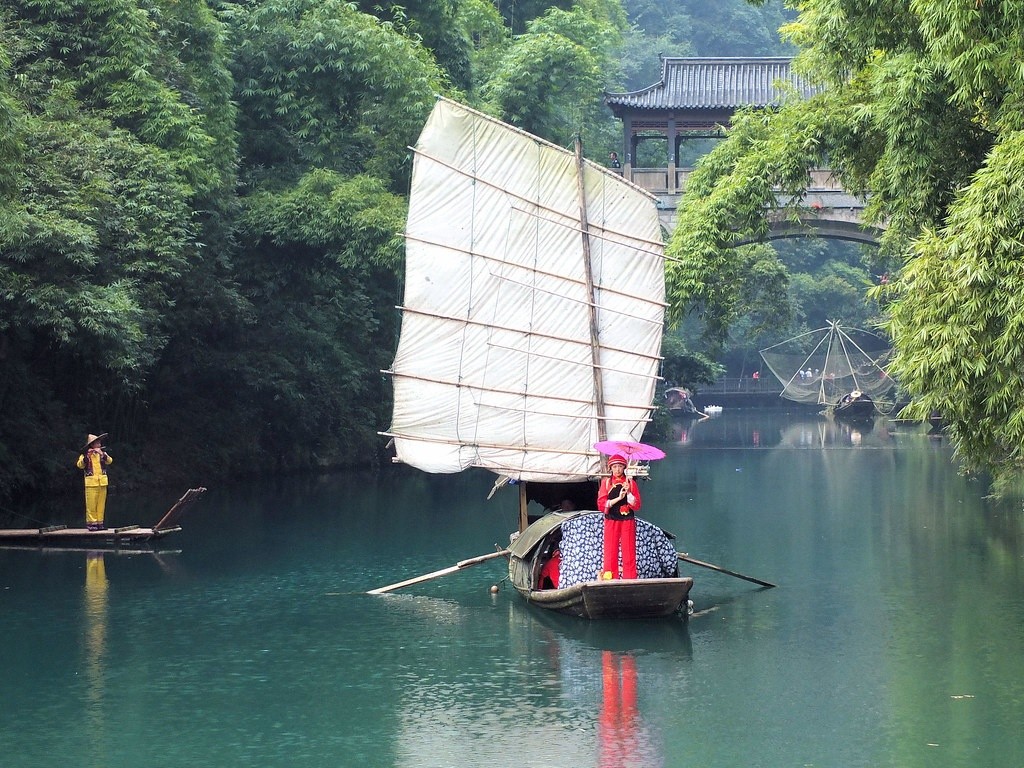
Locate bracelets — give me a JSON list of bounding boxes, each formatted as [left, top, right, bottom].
[[610, 500, 613, 507]]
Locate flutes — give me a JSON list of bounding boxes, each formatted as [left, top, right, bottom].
[[88, 446, 106, 452]]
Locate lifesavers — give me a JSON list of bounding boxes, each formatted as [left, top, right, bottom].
[[851, 391, 861, 398]]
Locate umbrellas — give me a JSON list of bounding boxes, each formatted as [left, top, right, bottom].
[[593, 440, 667, 492]]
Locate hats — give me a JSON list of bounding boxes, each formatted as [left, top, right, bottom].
[[608, 454, 627, 469], [81, 433, 108, 449]]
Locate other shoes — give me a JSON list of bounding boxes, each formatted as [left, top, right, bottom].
[[88, 525, 98, 531], [98, 524, 108, 530]]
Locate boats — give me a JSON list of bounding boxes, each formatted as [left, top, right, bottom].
[[664, 386, 694, 417], [832, 392, 876, 420], [0, 486, 207, 554]]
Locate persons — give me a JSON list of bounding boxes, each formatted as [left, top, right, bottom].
[[77, 433, 113, 532], [845, 391, 864, 402], [595, 648, 640, 768], [752, 371, 759, 392], [596, 454, 641, 579], [538, 534, 562, 589], [792, 368, 836, 391], [667, 388, 688, 409]]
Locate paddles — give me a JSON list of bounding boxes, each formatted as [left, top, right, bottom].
[[367, 549, 511, 594], [695, 410, 709, 416], [678, 555, 776, 587], [697, 416, 710, 423]]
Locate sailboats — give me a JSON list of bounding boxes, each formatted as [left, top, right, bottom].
[[377, 92, 693, 621]]
